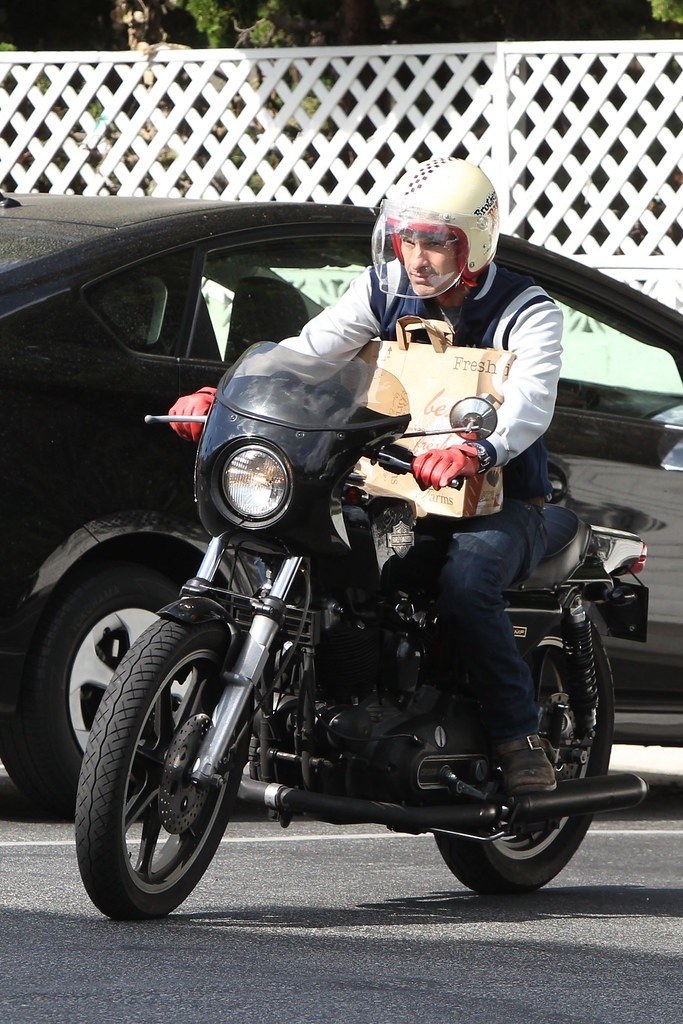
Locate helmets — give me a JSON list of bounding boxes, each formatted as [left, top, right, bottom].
[[382, 157, 499, 281]]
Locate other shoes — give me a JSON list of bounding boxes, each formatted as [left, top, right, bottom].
[[497, 734, 557, 795]]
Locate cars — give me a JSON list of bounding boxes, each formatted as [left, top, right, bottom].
[[0, 169, 683, 816]]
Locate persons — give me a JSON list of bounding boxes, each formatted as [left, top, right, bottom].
[[164, 160, 564, 793]]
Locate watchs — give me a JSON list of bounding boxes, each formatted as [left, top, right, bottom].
[[467, 442, 490, 475]]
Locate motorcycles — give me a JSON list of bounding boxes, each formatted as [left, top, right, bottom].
[[75, 341, 654, 929]]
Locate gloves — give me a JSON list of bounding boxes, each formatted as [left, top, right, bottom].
[[168, 386, 217, 442], [412, 438, 497, 491]]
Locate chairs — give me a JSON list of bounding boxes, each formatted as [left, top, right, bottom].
[[105, 276, 168, 347], [223, 278, 310, 363]]
